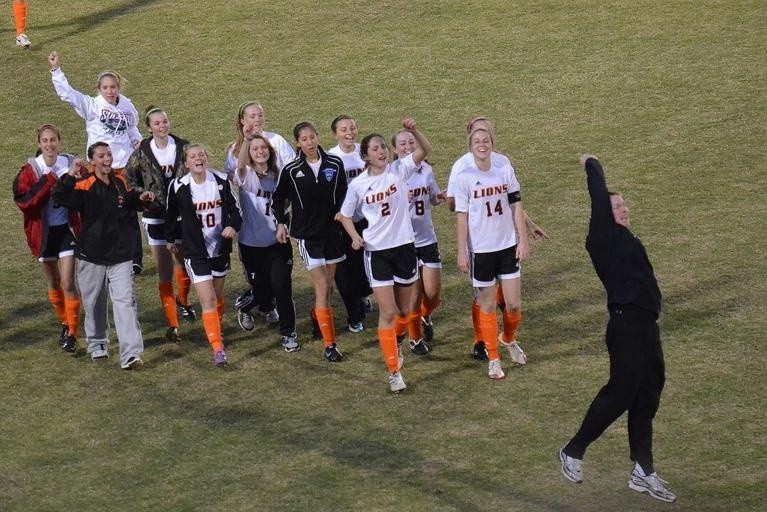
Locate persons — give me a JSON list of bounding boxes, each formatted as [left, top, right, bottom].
[[272, 122, 349, 362], [163, 144, 243, 367], [325, 114, 374, 333], [224, 100, 297, 326], [390, 130, 446, 369], [124, 105, 197, 343], [48, 50, 143, 275], [339, 111, 433, 392], [454, 129, 529, 380], [446, 116, 550, 360], [232, 114, 301, 353], [12, 124, 81, 352], [559, 152, 678, 504], [52, 142, 156, 370], [12, 0, 32, 47]]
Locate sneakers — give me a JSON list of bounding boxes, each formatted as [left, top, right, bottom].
[[348, 321, 364, 333], [167, 327, 178, 340], [260, 308, 279, 326], [628, 469, 677, 503], [14, 35, 31, 47], [388, 372, 407, 392], [236, 296, 255, 331], [311, 310, 323, 341], [62, 336, 79, 353], [133, 269, 142, 274], [213, 349, 227, 367], [488, 359, 504, 379], [558, 442, 584, 483], [474, 341, 488, 361], [421, 315, 434, 342], [91, 345, 109, 361], [409, 338, 428, 355], [362, 297, 372, 309], [324, 343, 345, 363], [59, 326, 68, 345], [280, 332, 300, 352], [176, 296, 198, 324], [120, 358, 143, 369], [498, 332, 528, 364], [398, 352, 403, 370]]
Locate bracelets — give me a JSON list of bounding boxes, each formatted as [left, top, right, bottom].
[[243, 137, 253, 143]]
